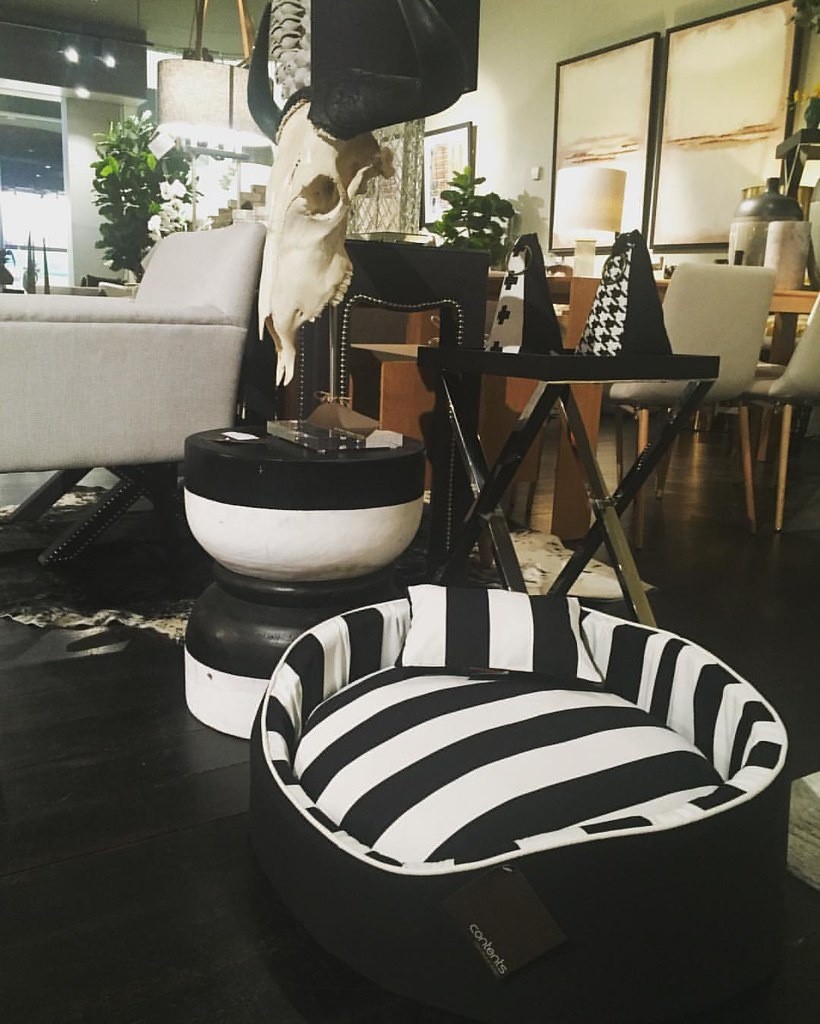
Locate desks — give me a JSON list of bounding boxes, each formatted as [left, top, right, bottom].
[[177, 422, 431, 740], [416, 347, 722, 631]]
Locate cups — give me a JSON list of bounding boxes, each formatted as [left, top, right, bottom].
[[763, 221, 813, 289]]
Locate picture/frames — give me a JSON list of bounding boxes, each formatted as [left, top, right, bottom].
[[649, 1, 802, 251], [420, 121, 474, 230], [547, 31, 659, 258]]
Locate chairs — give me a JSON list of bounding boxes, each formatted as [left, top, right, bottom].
[[731, 288, 820, 531], [3, 215, 262, 565], [608, 259, 778, 553]]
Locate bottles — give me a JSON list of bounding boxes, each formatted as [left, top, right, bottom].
[[728, 177, 803, 268]]
